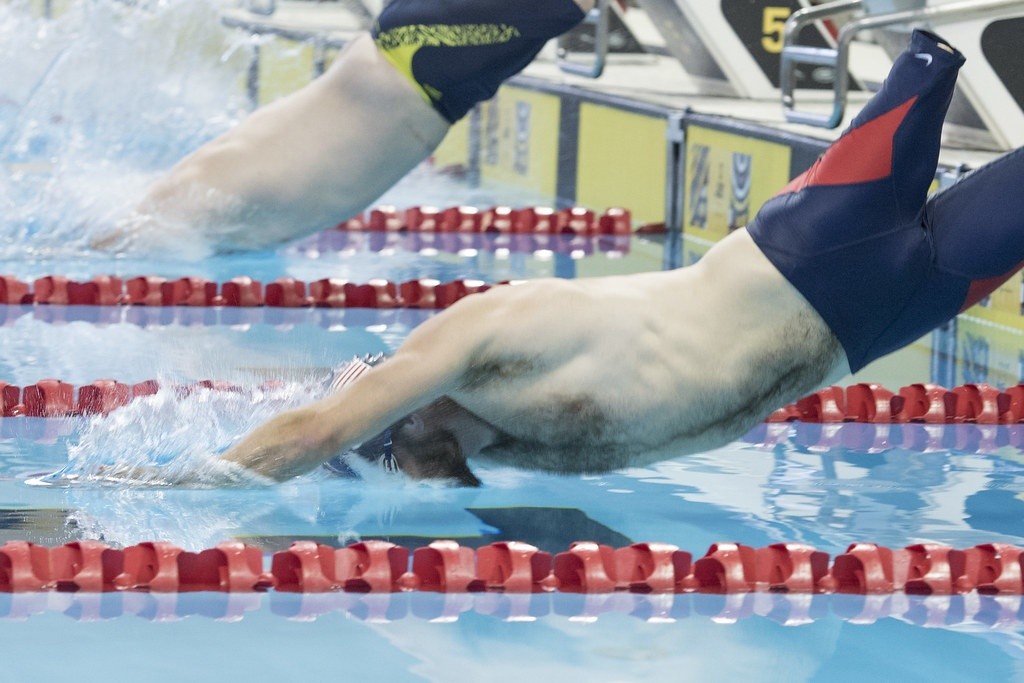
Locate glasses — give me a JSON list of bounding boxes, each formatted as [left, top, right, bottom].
[[377, 425, 401, 481]]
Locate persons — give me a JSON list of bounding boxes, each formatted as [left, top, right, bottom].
[[82, 0, 598, 262], [202, 28, 1023, 481]]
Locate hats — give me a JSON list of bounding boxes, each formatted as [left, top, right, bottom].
[[317, 355, 383, 479]]
